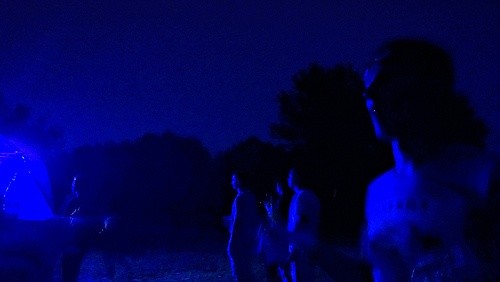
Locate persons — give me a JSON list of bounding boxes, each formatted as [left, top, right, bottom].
[[223, 165, 264, 282], [358, 36, 499, 282], [275, 178, 292, 221], [278, 163, 321, 282]]
